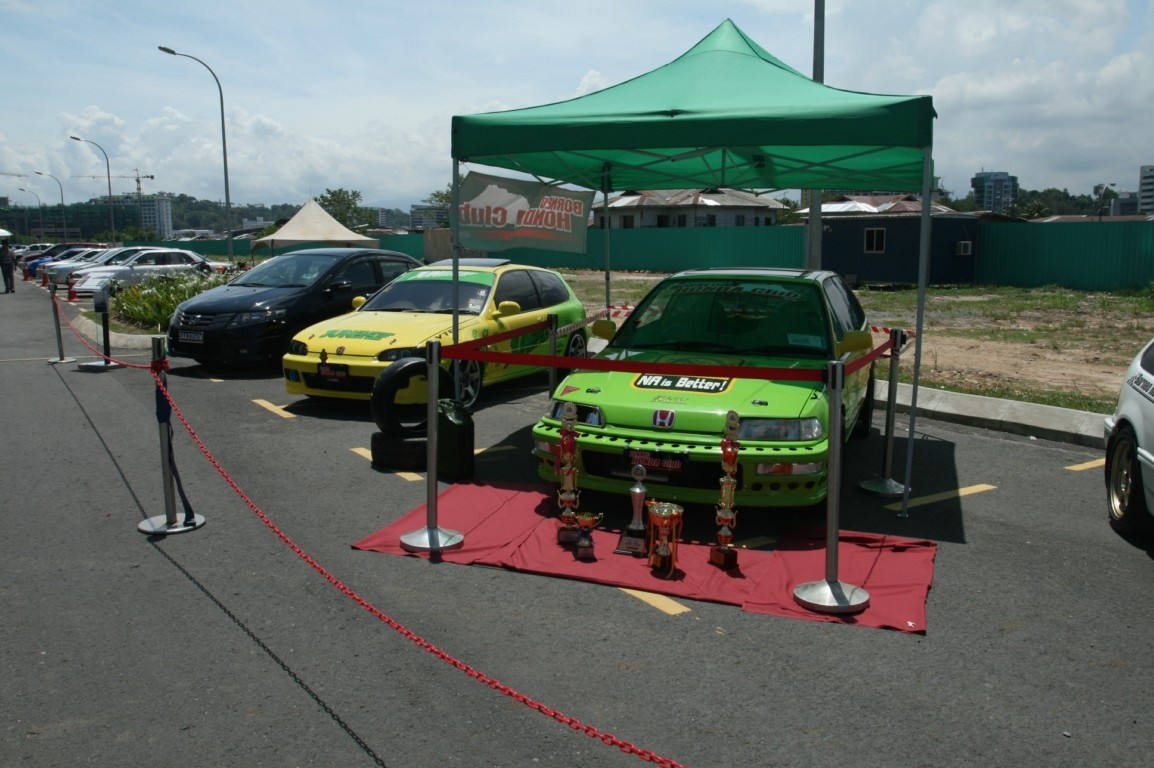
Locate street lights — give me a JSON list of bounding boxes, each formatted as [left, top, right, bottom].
[[156, 44, 238, 268], [70, 135, 117, 249], [34, 171, 69, 244], [17, 186, 44, 247]]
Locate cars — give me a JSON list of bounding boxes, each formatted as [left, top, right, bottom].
[[1099, 335, 1153, 537], [5, 239, 163, 283], [278, 255, 591, 419], [166, 247, 435, 377], [532, 264, 877, 521], [67, 249, 238, 298]]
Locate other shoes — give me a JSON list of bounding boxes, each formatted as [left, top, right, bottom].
[[11, 289, 15, 293], [5, 289, 10, 293]]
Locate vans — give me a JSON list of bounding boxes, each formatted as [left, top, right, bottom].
[[172, 229, 214, 242]]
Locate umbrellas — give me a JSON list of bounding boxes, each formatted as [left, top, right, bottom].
[[0, 229, 13, 236]]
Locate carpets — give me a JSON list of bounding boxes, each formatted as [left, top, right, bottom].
[[351, 455, 938, 635]]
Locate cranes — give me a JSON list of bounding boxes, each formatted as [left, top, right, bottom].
[[72, 168, 154, 233]]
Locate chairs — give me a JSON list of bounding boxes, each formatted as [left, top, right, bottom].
[[665, 297, 724, 342], [762, 299, 826, 342]]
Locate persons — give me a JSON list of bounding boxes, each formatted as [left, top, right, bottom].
[[200, 262, 211, 275], [0, 240, 18, 294]]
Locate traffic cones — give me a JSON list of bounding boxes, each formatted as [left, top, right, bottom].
[[40, 265, 52, 286], [61, 270, 81, 302], [21, 262, 33, 280]]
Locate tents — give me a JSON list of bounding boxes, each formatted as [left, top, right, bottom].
[[451, 18, 939, 518], [251, 199, 380, 268]]
